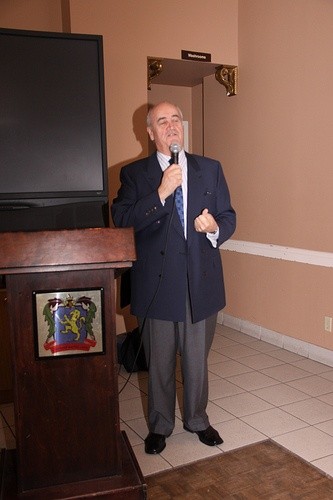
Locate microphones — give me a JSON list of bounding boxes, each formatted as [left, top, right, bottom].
[[169, 143, 180, 166]]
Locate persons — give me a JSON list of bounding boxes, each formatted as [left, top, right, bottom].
[[110, 101, 237, 454]]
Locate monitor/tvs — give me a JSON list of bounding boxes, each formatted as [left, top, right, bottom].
[[0, 28, 110, 211]]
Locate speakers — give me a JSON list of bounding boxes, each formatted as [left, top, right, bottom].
[[118, 327, 146, 372]]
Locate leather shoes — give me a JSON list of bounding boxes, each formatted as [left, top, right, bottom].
[[145, 431, 172, 454], [183, 424, 223, 446]]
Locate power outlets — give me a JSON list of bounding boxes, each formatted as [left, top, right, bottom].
[[324, 316, 332, 333]]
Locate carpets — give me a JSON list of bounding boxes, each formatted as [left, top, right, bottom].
[[144, 439, 333, 500]]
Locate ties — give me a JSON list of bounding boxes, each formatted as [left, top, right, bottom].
[[169, 159, 183, 230]]
